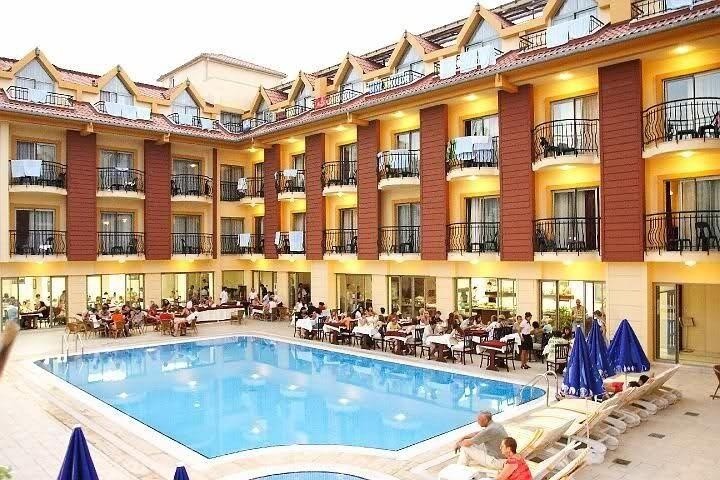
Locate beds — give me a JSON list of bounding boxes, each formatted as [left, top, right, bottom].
[[65, 308, 199, 338]]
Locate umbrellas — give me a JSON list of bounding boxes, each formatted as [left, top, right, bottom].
[[561, 319, 650, 446], [173, 467, 189, 480], [57, 427, 99, 480]]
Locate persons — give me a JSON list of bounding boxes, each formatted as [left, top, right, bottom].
[[387, 307, 552, 370], [460, 282, 497, 304], [455, 411, 534, 480], [342, 284, 387, 350], [188, 280, 215, 309], [571, 298, 605, 339], [220, 286, 234, 306], [147, 298, 195, 337], [543, 325, 572, 374], [290, 282, 330, 340], [241, 284, 283, 322], [86, 287, 144, 337], [2, 289, 66, 327]]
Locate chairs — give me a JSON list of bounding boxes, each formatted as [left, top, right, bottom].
[[479, 230, 499, 252], [37, 237, 54, 256], [400, 232, 414, 253], [125, 238, 137, 254], [181, 239, 193, 253], [437, 364, 682, 480], [536, 228, 558, 252], [345, 235, 357, 253], [667, 222, 720, 251], [37, 306, 50, 328], [230, 299, 291, 325], [293, 309, 571, 374]]
